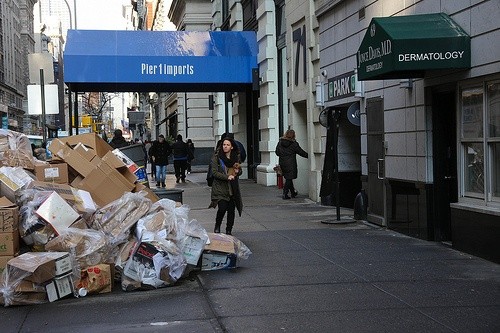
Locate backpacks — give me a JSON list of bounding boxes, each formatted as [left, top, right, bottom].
[[206, 159, 220, 186]]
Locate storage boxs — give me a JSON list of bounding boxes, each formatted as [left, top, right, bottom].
[[0, 129, 239, 307]]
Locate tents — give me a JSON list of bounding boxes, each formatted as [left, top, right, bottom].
[[63, 28, 259, 180]]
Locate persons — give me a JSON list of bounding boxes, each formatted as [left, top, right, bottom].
[[275, 130, 308, 200], [206, 132, 247, 236], [102, 129, 196, 188]]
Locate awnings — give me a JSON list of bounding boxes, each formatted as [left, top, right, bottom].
[[355, 11, 472, 82]]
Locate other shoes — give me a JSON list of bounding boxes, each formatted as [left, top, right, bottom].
[[226, 227, 231, 234], [214, 224, 220, 233], [282, 195, 290, 200], [162, 184, 166, 187], [156, 183, 160, 186], [292, 190, 297, 198], [176, 177, 180, 183], [182, 177, 185, 183]]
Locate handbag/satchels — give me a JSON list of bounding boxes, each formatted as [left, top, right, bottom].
[[273, 165, 283, 176]]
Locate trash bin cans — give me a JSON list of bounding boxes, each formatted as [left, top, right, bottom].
[[151, 188, 184, 206]]
